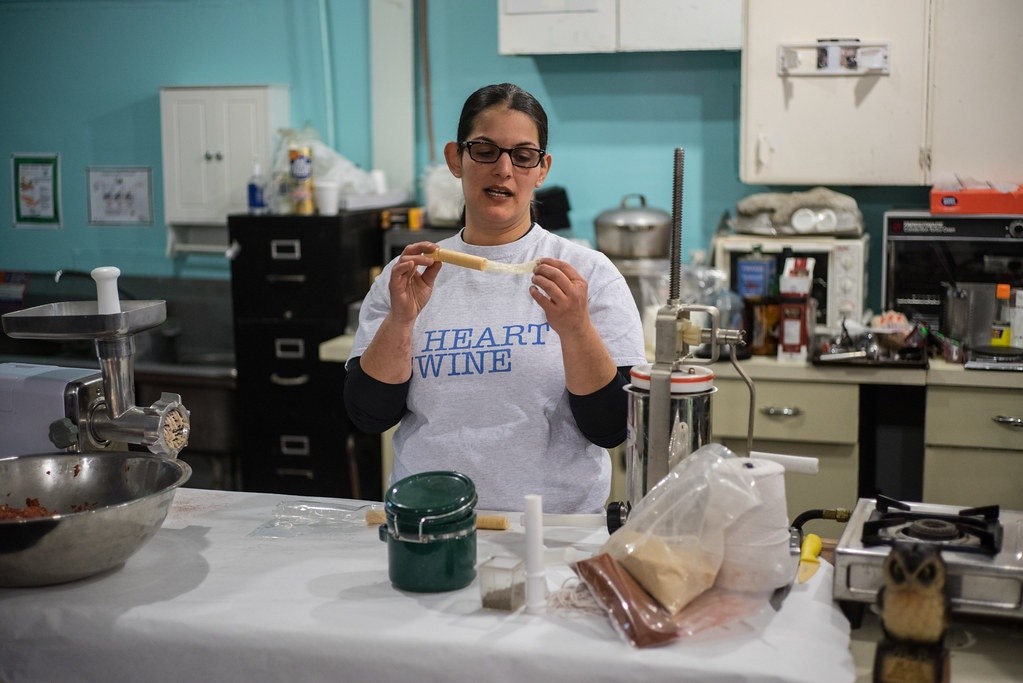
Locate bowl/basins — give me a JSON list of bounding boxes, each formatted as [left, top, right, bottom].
[[0, 452, 190, 587]]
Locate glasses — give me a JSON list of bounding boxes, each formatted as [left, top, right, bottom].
[[461, 140, 546, 168]]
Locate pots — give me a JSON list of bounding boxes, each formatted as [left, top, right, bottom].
[[593, 192, 671, 259], [939, 280, 1016, 356]]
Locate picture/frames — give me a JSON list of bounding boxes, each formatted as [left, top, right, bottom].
[[8, 151, 65, 232], [82, 164, 156, 229]]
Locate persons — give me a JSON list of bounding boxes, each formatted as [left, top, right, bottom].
[[343, 82, 647, 509]]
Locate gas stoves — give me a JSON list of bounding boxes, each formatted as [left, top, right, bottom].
[[833, 492, 1023, 630]]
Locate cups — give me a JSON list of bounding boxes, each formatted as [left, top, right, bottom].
[[311, 183, 339, 216]]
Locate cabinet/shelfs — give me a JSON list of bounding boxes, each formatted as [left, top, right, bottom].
[[159, 83, 290, 258], [494, 0, 1023, 196], [713, 375, 860, 543], [922, 385, 1023, 513], [228, 206, 411, 503]]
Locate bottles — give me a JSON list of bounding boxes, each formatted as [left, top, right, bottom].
[[990, 284, 1012, 347], [246, 164, 269, 216], [378, 470, 478, 594]]
